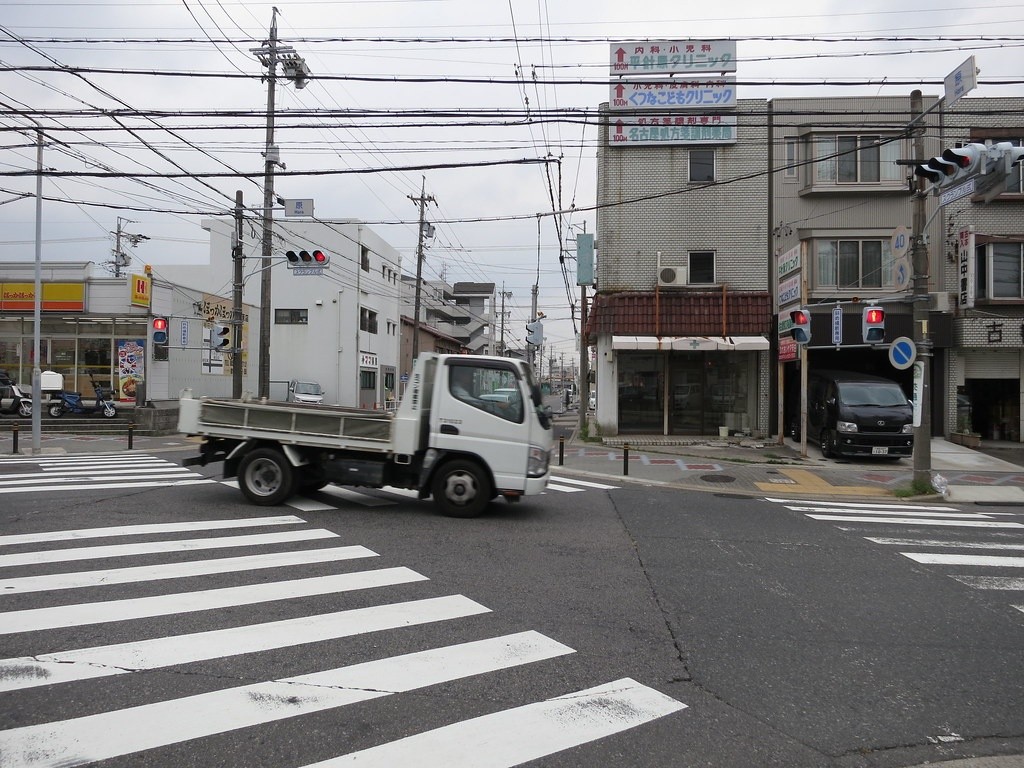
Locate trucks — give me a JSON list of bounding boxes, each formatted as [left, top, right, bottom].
[[182, 352, 570, 520]]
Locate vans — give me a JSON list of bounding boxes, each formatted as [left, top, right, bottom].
[[786, 368, 913, 460]]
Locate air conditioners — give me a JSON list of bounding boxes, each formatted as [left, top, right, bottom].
[[658, 265, 687, 286], [928, 292, 948, 311]]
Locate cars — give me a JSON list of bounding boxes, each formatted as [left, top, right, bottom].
[[477, 387, 518, 412]]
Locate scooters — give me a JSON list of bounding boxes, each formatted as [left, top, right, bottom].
[[43, 371, 117, 419], [0, 370, 32, 418]]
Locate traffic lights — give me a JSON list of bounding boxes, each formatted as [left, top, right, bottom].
[[1003, 141, 1024, 175], [862, 306, 886, 343], [152, 317, 168, 344], [285, 248, 329, 269], [213, 324, 229, 348], [790, 310, 811, 344], [914, 143, 989, 189]]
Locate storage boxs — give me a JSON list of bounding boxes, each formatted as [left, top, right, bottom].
[[41, 373, 64, 390]]
